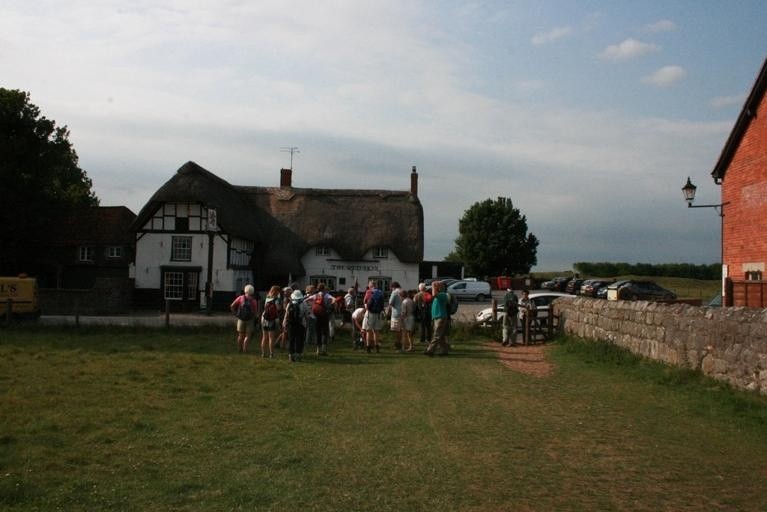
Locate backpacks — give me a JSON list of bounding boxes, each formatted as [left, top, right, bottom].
[[237, 295, 255, 321], [505, 294, 518, 317], [448, 293, 459, 315], [416, 293, 428, 312], [367, 290, 384, 314], [312, 293, 327, 317], [263, 297, 278, 321], [287, 301, 304, 327]]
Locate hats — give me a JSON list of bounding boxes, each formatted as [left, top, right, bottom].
[[336, 295, 344, 303], [290, 289, 304, 300]]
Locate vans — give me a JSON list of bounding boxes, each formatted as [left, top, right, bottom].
[[427, 278, 491, 303]]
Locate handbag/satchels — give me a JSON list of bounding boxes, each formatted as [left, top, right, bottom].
[[412, 299, 425, 322]]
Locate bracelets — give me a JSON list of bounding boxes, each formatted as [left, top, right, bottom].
[[231, 312, 236, 315]]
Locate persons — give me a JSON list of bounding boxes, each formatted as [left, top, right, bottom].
[[229, 283, 258, 355], [249, 285, 261, 341], [260, 277, 458, 364], [516, 289, 536, 345], [501, 287, 520, 347]]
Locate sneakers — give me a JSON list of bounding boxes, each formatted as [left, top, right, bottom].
[[261, 352, 274, 359], [287, 353, 304, 365], [420, 340, 449, 356], [502, 340, 515, 348], [316, 344, 329, 360], [393, 344, 414, 354]]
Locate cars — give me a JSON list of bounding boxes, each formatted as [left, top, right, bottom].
[[543, 275, 617, 298], [597, 279, 677, 303], [476, 292, 577, 334]]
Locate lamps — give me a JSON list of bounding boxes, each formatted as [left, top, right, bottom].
[[681, 176, 730, 217]]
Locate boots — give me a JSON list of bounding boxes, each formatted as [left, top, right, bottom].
[[352, 341, 380, 354]]
